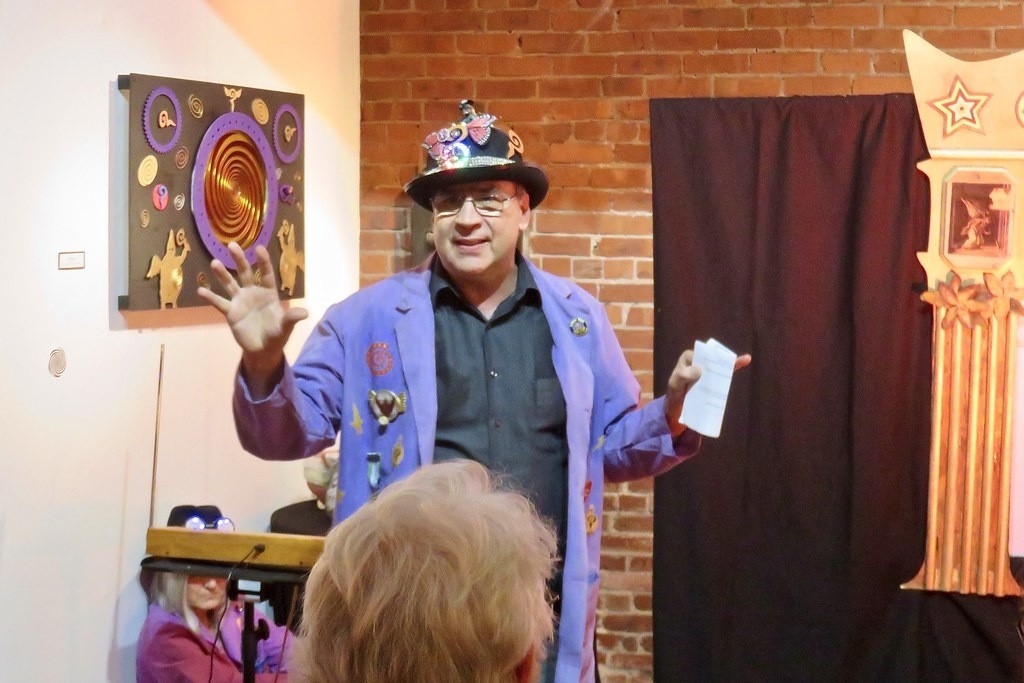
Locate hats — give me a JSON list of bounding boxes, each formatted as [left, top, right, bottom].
[[402, 100, 551, 212], [166, 505, 237, 531]]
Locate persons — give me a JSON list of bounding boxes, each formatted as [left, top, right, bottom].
[[196, 97, 764, 683], [295, 459, 558, 683], [134, 504, 294, 683]]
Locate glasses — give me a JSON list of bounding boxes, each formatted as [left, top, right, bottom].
[[427, 186, 518, 216], [183, 517, 239, 535]]
